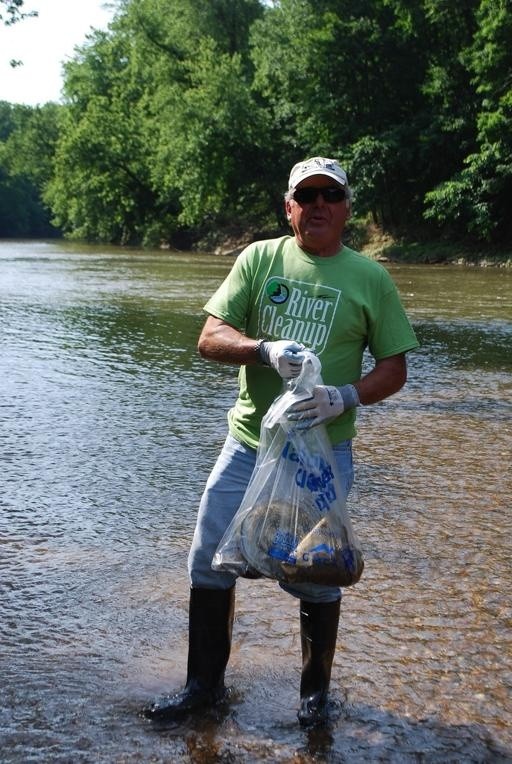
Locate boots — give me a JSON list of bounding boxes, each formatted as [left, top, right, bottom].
[[298, 600, 340, 724], [140, 585, 237, 722]]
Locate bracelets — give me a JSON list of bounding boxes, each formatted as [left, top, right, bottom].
[[254, 337, 270, 368]]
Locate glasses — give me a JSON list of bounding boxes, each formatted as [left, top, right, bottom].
[[289, 184, 351, 205]]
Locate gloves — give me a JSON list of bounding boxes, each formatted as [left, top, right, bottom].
[[288, 382, 360, 430], [260, 340, 305, 379]]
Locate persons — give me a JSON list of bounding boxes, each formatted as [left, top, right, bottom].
[[139, 156, 422, 730]]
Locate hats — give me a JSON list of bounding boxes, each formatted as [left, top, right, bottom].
[[288, 156, 351, 191]]
